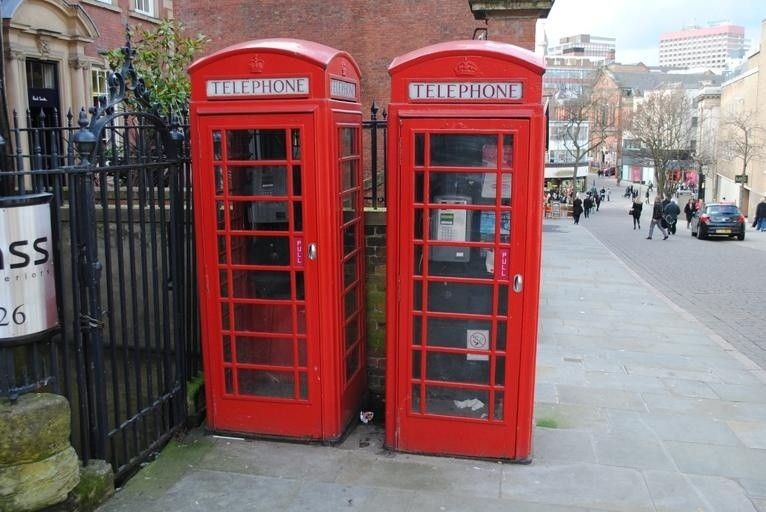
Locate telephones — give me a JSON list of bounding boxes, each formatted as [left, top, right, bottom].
[[239, 166, 297, 224], [417, 195, 473, 263]]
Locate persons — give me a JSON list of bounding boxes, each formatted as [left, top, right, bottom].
[[543, 165, 766, 232], [646, 196, 669, 240]]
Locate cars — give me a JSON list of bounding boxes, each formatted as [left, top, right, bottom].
[[691, 204, 746, 241], [603, 166, 614, 177]]
